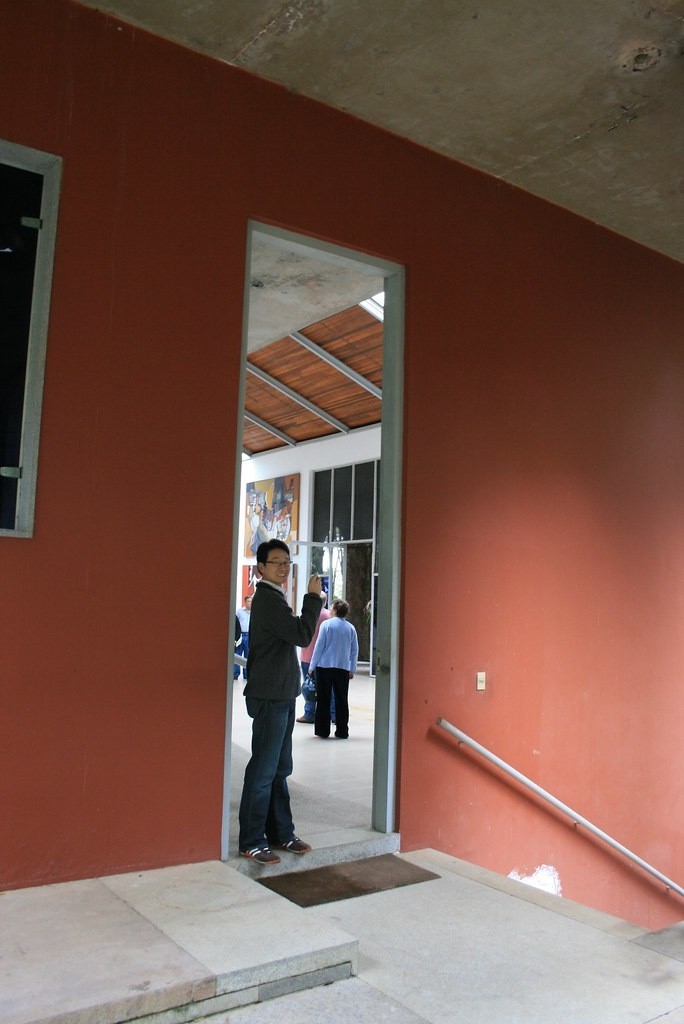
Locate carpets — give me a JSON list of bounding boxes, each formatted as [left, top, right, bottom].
[[256, 854, 442, 908]]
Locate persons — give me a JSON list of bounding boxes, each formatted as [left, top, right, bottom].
[[363, 601, 371, 624], [239, 539, 324, 864], [296, 590, 335, 723], [233, 595, 251, 680], [309, 600, 358, 739]]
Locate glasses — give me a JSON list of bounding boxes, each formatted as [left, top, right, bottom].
[[261, 559, 294, 568]]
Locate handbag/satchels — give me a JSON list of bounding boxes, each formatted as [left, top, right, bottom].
[[302, 673, 318, 702]]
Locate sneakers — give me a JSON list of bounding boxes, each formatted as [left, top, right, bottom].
[[239, 844, 280, 864], [268, 835, 311, 853]]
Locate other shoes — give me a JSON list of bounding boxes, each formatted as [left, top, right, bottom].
[[234, 675, 238, 679], [296, 715, 314, 724]]
